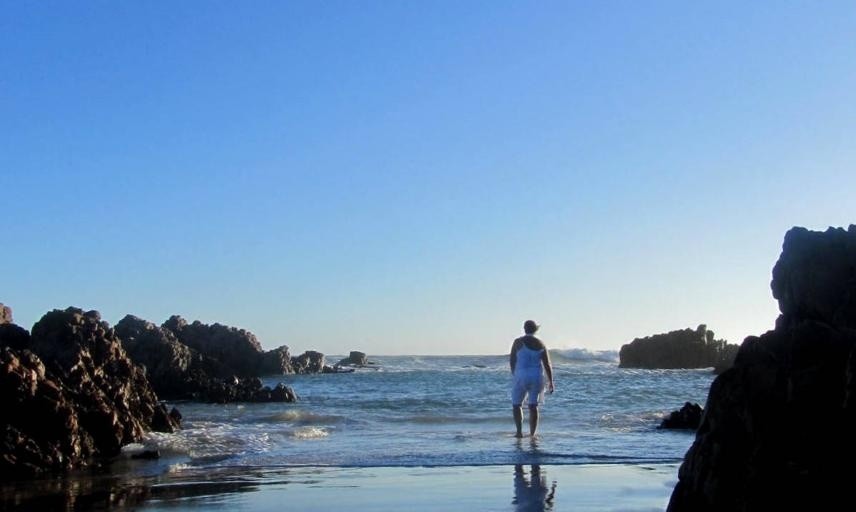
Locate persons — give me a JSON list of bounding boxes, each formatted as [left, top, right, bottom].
[[509, 319, 554, 438], [512, 437, 558, 512]]
